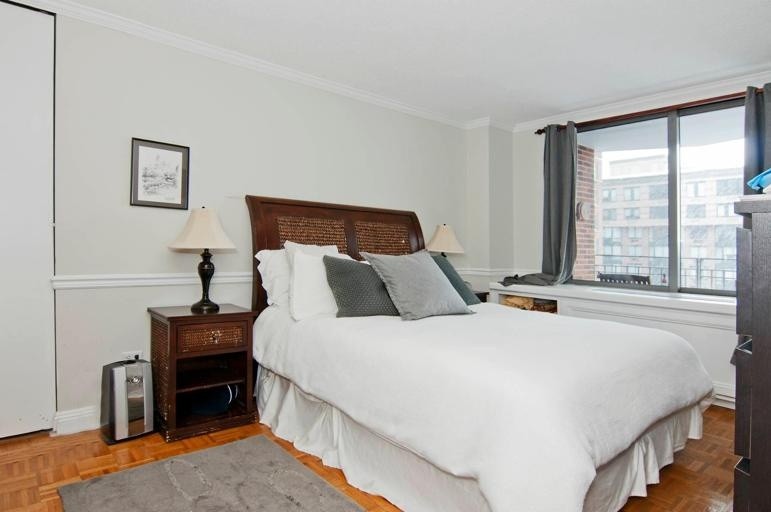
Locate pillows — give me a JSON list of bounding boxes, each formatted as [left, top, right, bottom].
[[254, 245, 340, 308], [322, 255, 401, 319], [429, 254, 484, 306], [284, 239, 372, 322], [358, 247, 478, 322]]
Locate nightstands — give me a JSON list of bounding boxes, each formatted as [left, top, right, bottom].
[[143, 301, 269, 444]]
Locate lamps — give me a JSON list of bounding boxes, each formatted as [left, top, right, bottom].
[[164, 205, 239, 314], [424, 223, 467, 261]]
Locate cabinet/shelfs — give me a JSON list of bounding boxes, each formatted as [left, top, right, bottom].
[[727, 196, 770, 512]]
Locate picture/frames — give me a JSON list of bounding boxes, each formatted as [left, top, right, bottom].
[[129, 136, 191, 209]]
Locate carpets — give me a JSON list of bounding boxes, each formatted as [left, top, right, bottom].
[[54, 433, 367, 512]]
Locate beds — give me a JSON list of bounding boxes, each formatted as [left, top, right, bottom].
[[245, 193, 716, 512]]
